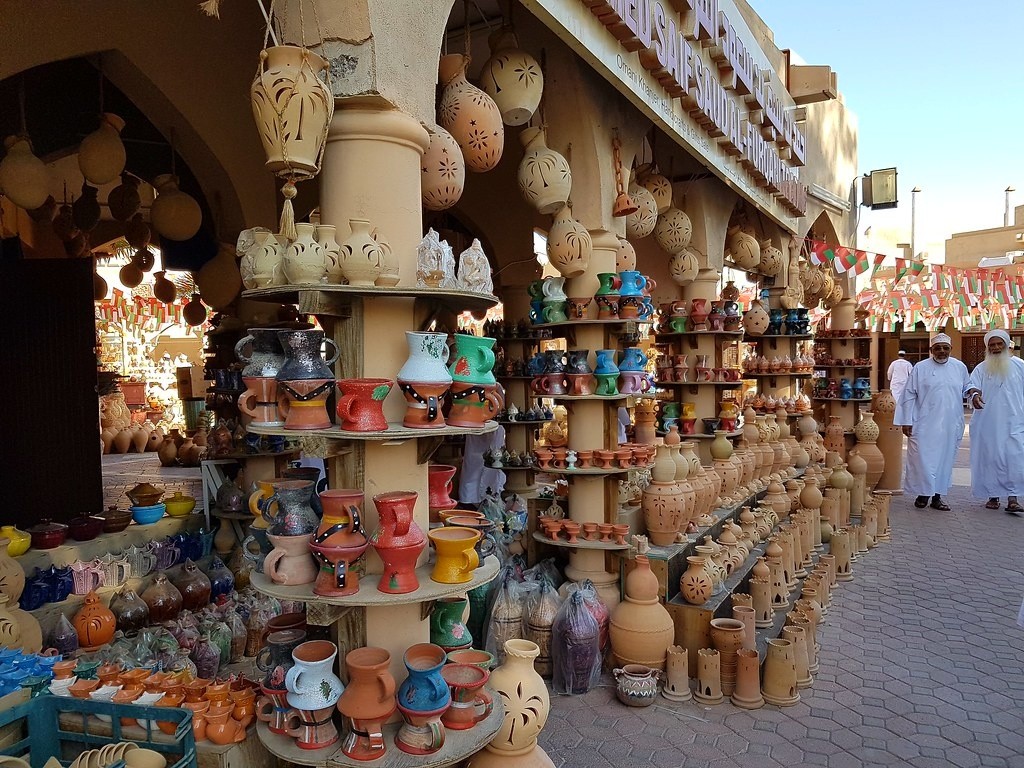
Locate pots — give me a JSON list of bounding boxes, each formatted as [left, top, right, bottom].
[[0, 482, 194, 554]]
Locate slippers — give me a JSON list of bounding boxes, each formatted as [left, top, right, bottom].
[[986, 500, 1000, 509], [930, 496, 950, 511], [914, 496, 930, 508], [1005, 500, 1024, 512]]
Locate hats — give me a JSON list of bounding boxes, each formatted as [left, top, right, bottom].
[[1009, 340, 1015, 348], [931, 333, 951, 347], [984, 330, 1010, 346], [899, 351, 905, 354]]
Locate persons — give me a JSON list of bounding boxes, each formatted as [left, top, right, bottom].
[[891, 333, 983, 511], [967, 328, 1024, 513], [886, 350, 914, 405]]
